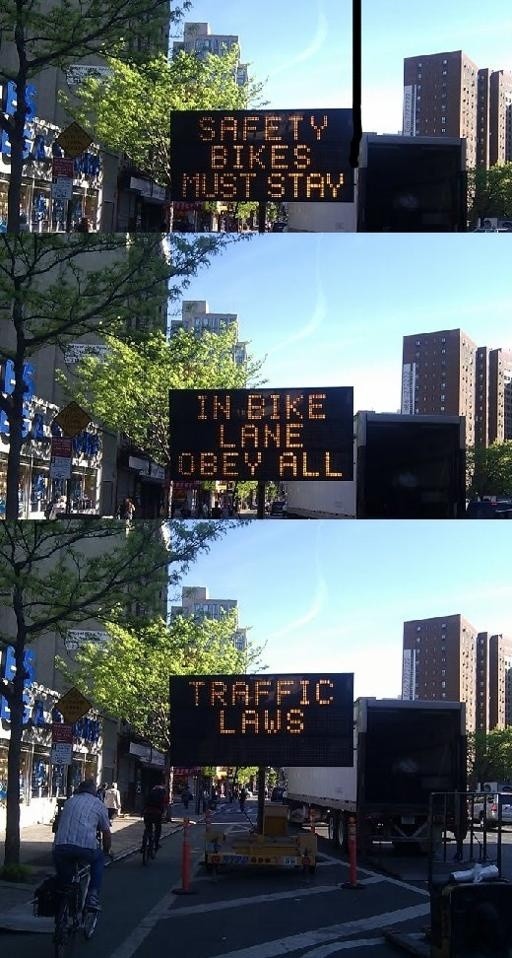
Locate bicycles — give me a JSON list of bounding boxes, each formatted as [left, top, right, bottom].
[[46, 850, 114, 958], [139, 830, 160, 865]]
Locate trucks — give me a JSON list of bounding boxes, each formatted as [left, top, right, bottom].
[[271, 697, 468, 856], [279, 410, 466, 519], [284, 132, 468, 232]]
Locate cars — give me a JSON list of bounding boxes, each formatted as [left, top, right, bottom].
[[468, 791, 512, 829]]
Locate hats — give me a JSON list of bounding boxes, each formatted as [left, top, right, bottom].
[[77, 779, 97, 795]]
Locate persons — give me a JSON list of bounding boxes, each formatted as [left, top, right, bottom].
[[179, 779, 250, 816], [52, 778, 111, 942], [77, 217, 92, 232], [97, 782, 109, 801], [103, 782, 122, 827], [0, 491, 273, 520], [451, 801, 469, 861], [139, 777, 172, 854], [20, 213, 30, 233]]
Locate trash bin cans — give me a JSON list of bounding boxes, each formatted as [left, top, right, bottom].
[[428, 874, 509, 957]]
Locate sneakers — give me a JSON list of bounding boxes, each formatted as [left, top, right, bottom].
[[85, 895, 103, 914]]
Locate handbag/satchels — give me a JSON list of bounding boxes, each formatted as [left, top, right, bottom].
[[32, 879, 60, 919]]
